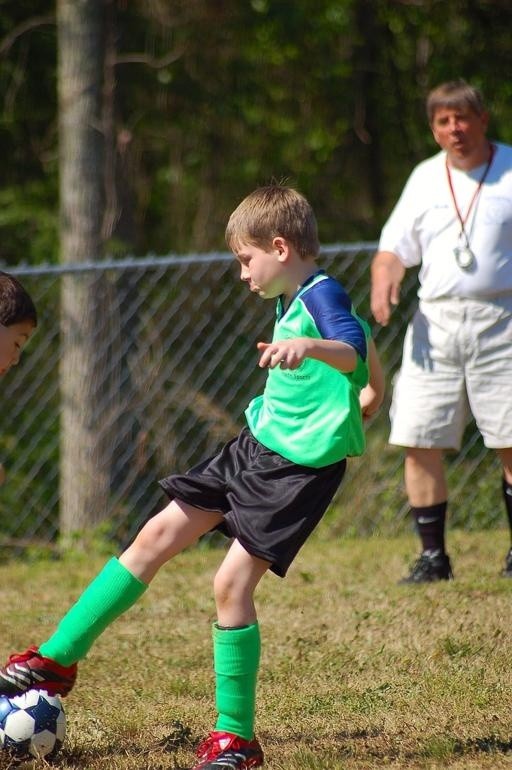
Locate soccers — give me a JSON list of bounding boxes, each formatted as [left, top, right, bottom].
[[1, 689, 67, 761]]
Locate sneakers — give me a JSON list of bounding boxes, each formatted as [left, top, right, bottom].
[[0, 645, 77, 697], [397, 549, 454, 584], [191, 731, 263, 770]]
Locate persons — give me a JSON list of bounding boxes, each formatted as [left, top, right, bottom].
[[370, 79, 512, 584], [0, 175, 387, 770], [1, 273, 37, 379]]
